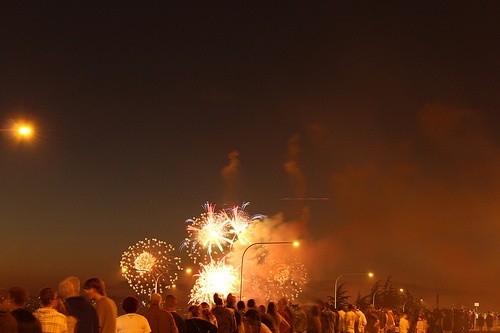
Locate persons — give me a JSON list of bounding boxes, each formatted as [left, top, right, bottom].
[[0, 276, 500, 333]]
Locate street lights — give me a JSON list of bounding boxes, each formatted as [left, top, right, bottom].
[[240, 241, 301, 301], [335, 273, 374, 310], [120, 266, 192, 294]]
